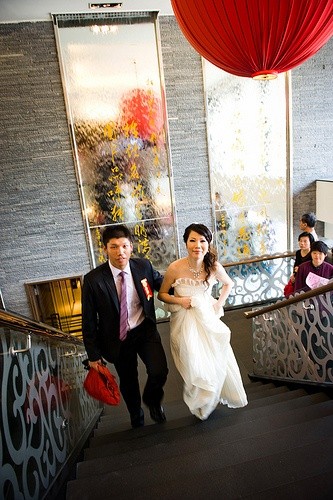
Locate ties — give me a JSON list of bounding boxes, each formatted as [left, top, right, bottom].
[[119, 270, 129, 341]]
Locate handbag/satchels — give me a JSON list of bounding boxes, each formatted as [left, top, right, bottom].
[[284, 280, 294, 298], [83, 362, 121, 407]]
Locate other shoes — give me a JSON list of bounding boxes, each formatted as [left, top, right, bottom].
[[131, 415, 143, 429], [150, 403, 167, 425]]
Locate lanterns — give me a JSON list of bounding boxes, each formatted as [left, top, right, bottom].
[[170, 0, 333, 83]]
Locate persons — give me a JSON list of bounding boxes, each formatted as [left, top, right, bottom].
[[293, 241, 333, 294], [156, 224, 247, 421], [292, 231, 317, 277], [298, 212, 319, 243], [79, 224, 179, 429]]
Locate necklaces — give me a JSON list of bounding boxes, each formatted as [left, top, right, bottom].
[[186, 255, 205, 281]]
